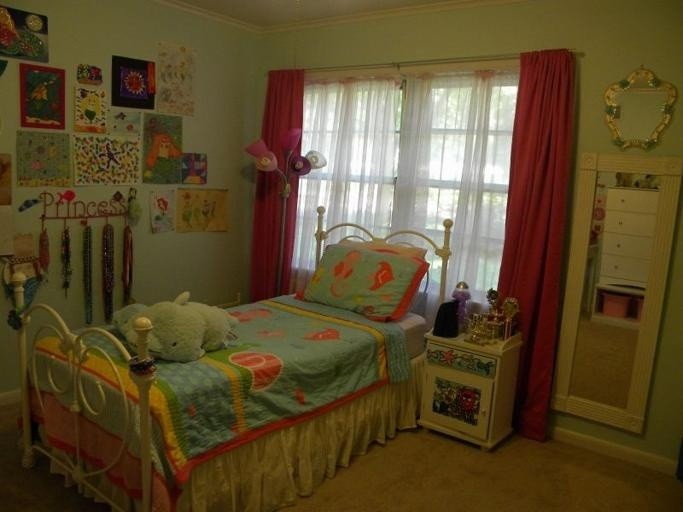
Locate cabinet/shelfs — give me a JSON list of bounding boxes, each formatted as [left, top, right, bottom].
[[591, 184, 657, 330]]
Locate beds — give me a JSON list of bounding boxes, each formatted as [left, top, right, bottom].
[[7, 207, 453, 510]]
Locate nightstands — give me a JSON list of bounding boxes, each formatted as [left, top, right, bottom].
[[417, 329, 523, 451]]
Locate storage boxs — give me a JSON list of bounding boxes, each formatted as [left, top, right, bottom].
[[604, 292, 643, 321]]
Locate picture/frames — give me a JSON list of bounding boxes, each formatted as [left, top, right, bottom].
[[112, 55, 155, 110], [20, 63, 65, 128]]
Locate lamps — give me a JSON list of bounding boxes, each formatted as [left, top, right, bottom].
[[242, 128, 327, 297]]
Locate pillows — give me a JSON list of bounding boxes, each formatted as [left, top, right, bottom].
[[341, 238, 428, 260], [303, 245, 420, 318]]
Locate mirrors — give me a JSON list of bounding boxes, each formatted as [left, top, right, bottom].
[[547, 152, 683, 434]]
